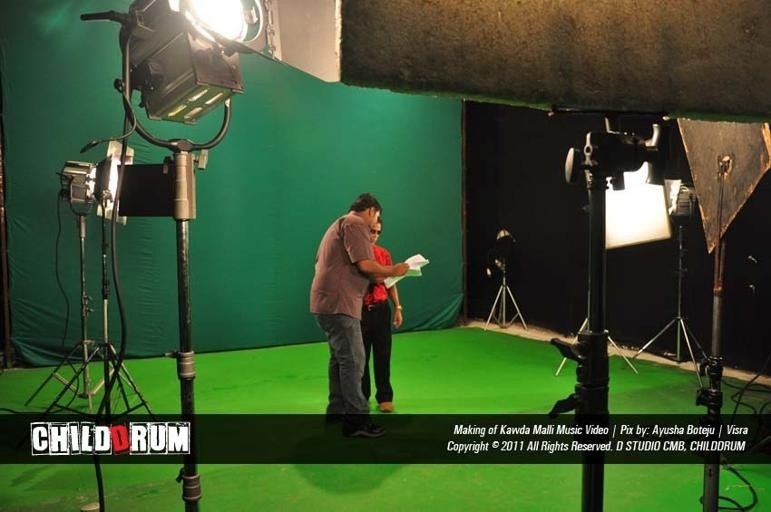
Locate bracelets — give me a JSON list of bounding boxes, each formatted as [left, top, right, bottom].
[[395, 304, 404, 311]]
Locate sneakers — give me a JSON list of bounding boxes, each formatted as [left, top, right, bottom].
[[343, 422, 386, 437], [380, 402, 393, 412]]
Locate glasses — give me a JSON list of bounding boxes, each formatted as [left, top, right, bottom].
[[370, 229, 381, 234]]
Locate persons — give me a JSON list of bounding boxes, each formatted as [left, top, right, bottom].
[[359, 218, 405, 416], [309, 192, 411, 439]]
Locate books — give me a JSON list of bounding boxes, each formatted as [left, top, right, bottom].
[[383, 253, 431, 290]]
